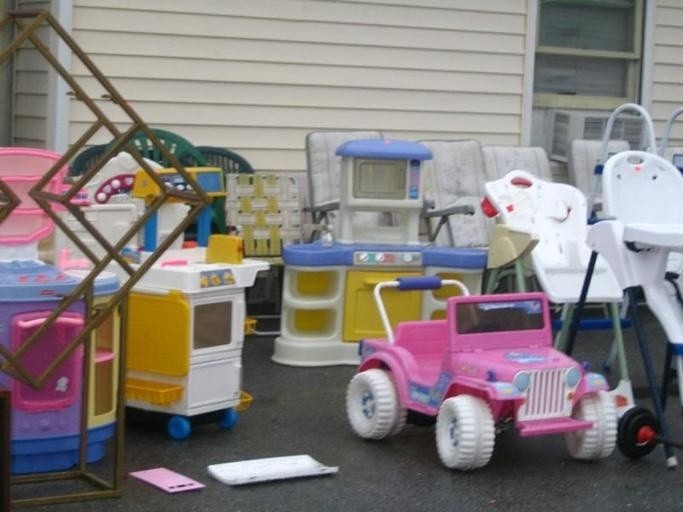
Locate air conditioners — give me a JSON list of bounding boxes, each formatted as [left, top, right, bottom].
[[548, 108, 642, 162]]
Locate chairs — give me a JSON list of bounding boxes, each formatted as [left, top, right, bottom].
[[305, 130, 683, 470]]
